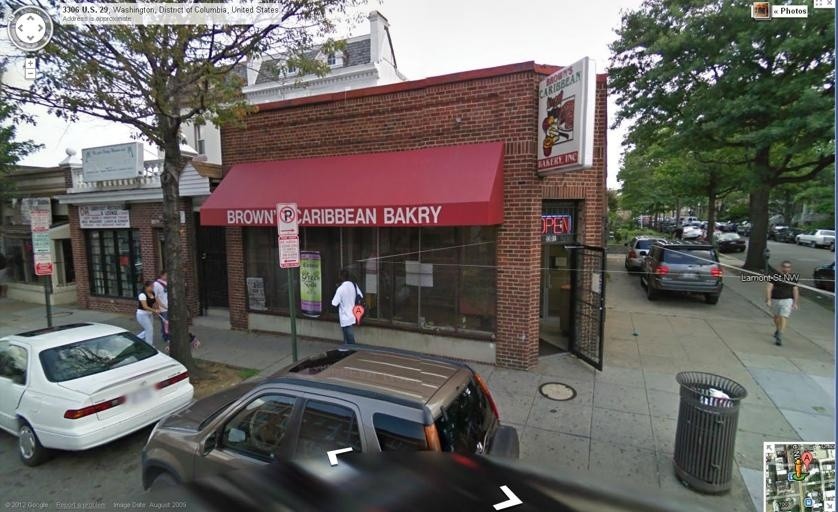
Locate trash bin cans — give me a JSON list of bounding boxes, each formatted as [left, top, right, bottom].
[[673, 371, 747, 495]]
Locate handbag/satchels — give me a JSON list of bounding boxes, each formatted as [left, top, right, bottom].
[[352, 296, 366, 325]]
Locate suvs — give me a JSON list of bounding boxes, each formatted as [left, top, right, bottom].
[[139, 343, 519, 506], [638, 239, 723, 304]]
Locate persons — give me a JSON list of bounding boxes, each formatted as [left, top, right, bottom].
[[768, 223, 778, 242], [766, 260, 797, 347], [329, 271, 364, 344], [151, 270, 169, 342], [136, 281, 162, 345], [671, 222, 684, 241]]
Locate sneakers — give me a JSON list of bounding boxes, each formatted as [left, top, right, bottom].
[[773, 330, 780, 339], [776, 338, 782, 346]]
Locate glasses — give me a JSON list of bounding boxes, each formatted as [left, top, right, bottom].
[[784, 265, 791, 268]]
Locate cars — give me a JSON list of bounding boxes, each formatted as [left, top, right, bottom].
[[812, 260, 835, 292], [623, 236, 667, 271], [0, 321, 194, 467], [636, 215, 834, 253]]
